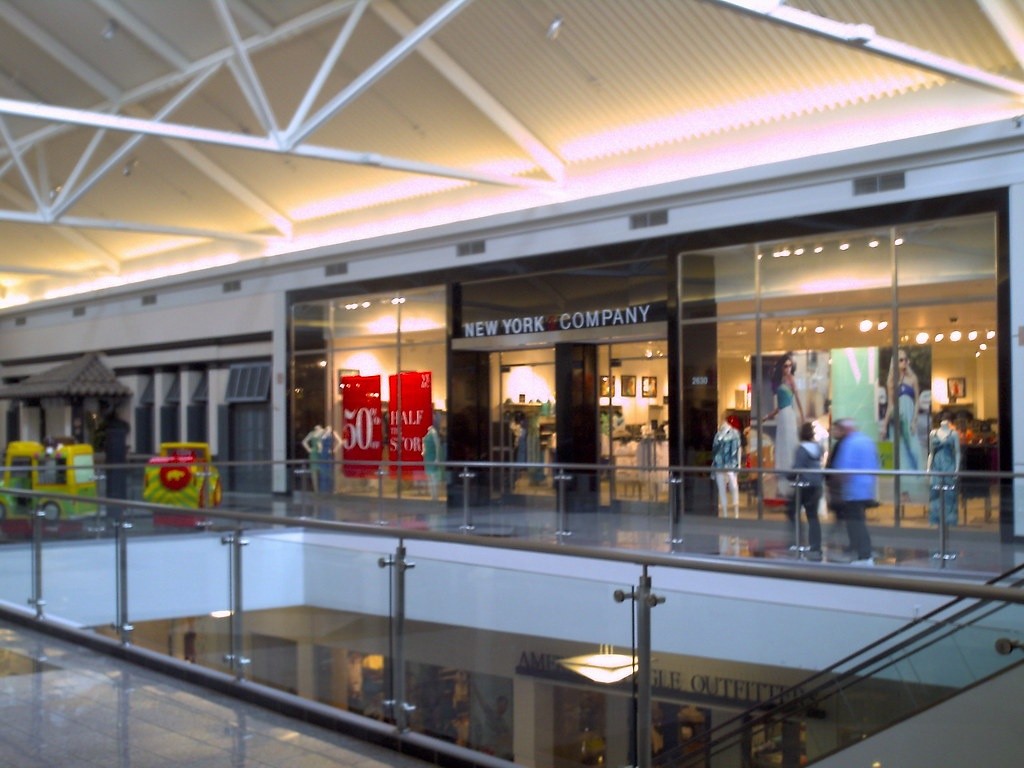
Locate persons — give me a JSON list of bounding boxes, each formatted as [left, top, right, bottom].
[[421, 425, 441, 499], [762, 355, 804, 497], [476, 692, 507, 743], [825, 420, 880, 566], [712, 423, 741, 518], [95, 411, 130, 518], [615, 411, 623, 428], [927, 421, 961, 526], [786, 423, 823, 556], [319, 426, 342, 492], [302, 425, 323, 491], [880, 350, 922, 495]]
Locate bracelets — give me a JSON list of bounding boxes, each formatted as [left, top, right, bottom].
[[766, 415, 768, 418]]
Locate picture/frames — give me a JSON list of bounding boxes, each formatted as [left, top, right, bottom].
[[641, 376, 657, 398], [600, 375, 615, 396], [620, 375, 637, 397], [946, 378, 965, 397]]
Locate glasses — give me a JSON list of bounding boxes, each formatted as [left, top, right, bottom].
[[898, 358, 907, 362], [783, 364, 792, 367]]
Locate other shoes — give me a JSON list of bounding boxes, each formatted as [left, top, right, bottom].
[[844, 545, 857, 553], [804, 550, 822, 556], [851, 558, 874, 566]]
